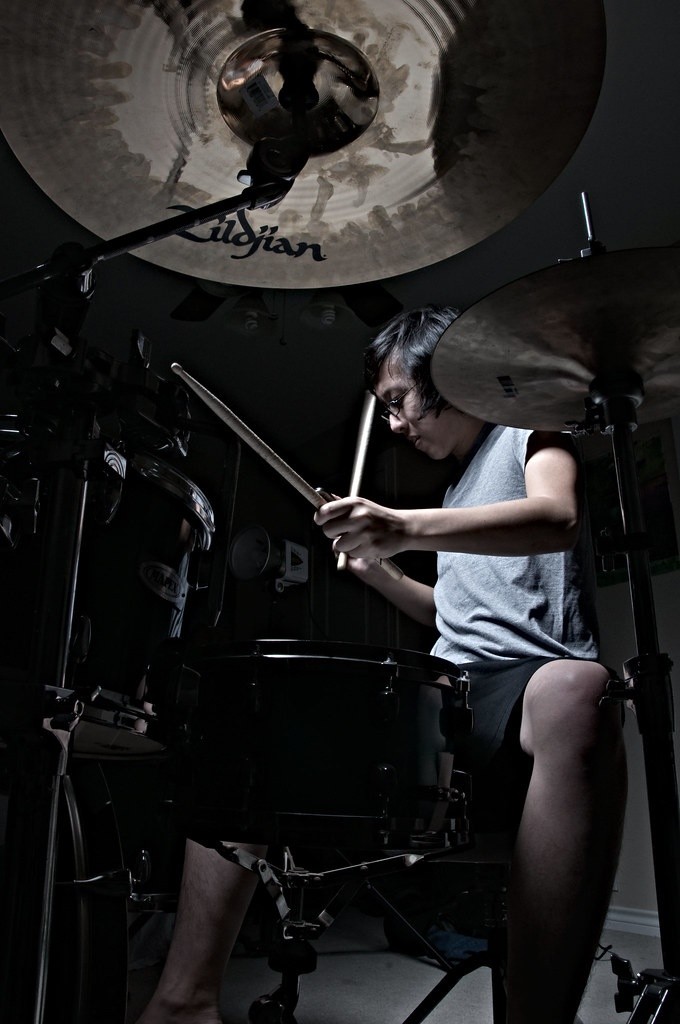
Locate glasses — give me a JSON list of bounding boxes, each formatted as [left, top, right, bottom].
[[380, 381, 418, 424]]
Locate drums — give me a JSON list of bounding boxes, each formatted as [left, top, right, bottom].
[[159, 637, 477, 852], [0, 414, 218, 764], [0, 758, 130, 1024]]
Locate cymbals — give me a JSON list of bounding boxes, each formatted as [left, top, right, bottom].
[[0, 1, 610, 292], [426, 244, 679, 433]]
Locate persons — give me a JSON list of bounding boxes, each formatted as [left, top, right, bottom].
[[138, 301, 628, 1024]]
